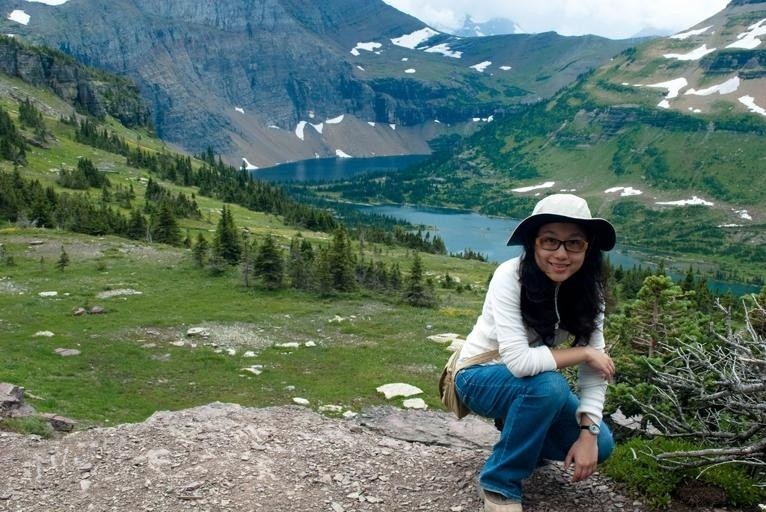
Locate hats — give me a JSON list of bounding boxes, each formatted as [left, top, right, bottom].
[[507, 194, 616, 251]]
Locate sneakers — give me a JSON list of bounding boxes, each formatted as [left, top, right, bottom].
[[475, 474, 524, 512], [495, 416, 504, 432]]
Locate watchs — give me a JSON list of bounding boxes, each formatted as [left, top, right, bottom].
[[578, 422, 601, 436]]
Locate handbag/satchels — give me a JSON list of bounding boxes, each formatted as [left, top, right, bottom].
[[439, 350, 470, 420]]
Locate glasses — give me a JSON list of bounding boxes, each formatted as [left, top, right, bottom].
[[536, 236, 589, 253]]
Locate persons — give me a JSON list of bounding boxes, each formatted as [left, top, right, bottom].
[[447, 189, 620, 512]]
